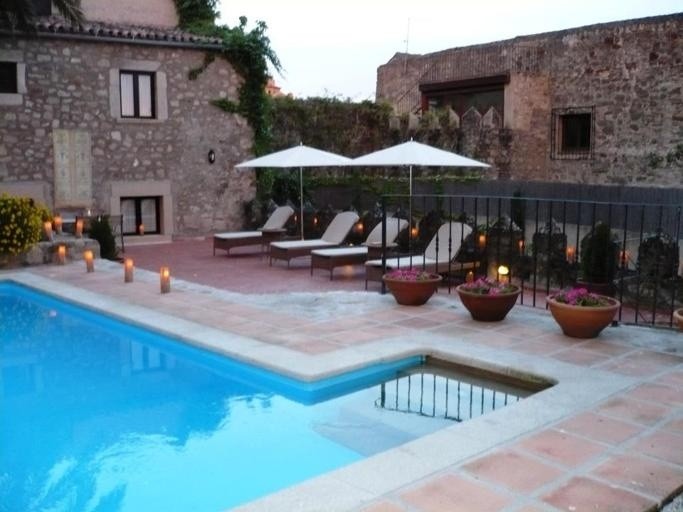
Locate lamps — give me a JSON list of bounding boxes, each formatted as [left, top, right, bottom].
[[209, 149, 216, 163]]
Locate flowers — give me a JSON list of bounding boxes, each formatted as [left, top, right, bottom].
[[462, 276, 518, 294], [389, 270, 431, 280], [555, 289, 611, 306]]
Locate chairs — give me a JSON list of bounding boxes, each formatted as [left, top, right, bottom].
[[365, 221, 480, 294], [310, 217, 409, 280], [268, 212, 360, 267], [213, 205, 294, 258]]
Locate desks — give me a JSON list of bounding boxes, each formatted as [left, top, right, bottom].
[[257, 227, 287, 258], [367, 241, 398, 262]]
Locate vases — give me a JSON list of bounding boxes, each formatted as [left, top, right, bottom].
[[544, 288, 622, 338], [455, 276, 523, 322], [382, 270, 443, 305]]
